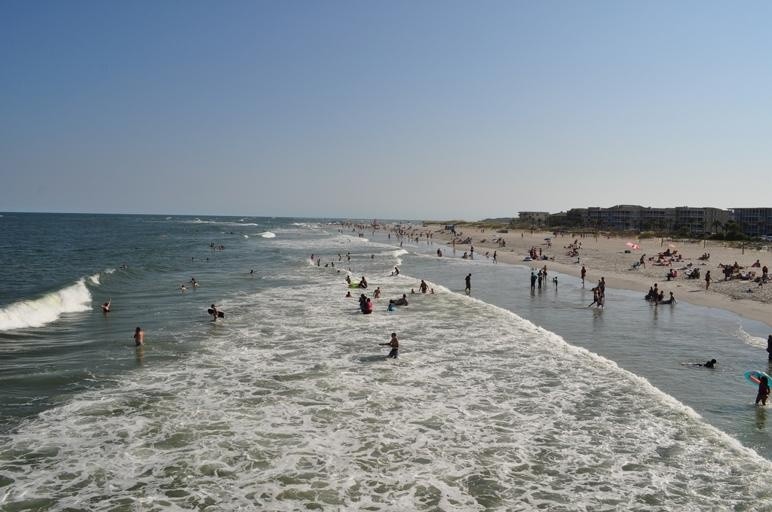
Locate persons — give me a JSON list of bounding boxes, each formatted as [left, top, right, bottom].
[[179, 241, 254, 321], [337, 222, 433, 247], [628, 248, 771, 306], [767, 335, 771, 363], [695, 358, 717, 369], [99, 297, 112, 312], [310, 251, 434, 314], [464, 272, 471, 294], [756, 375, 771, 406], [134, 326, 143, 346], [386, 333, 399, 359], [530, 230, 606, 308], [437, 229, 523, 263]]
